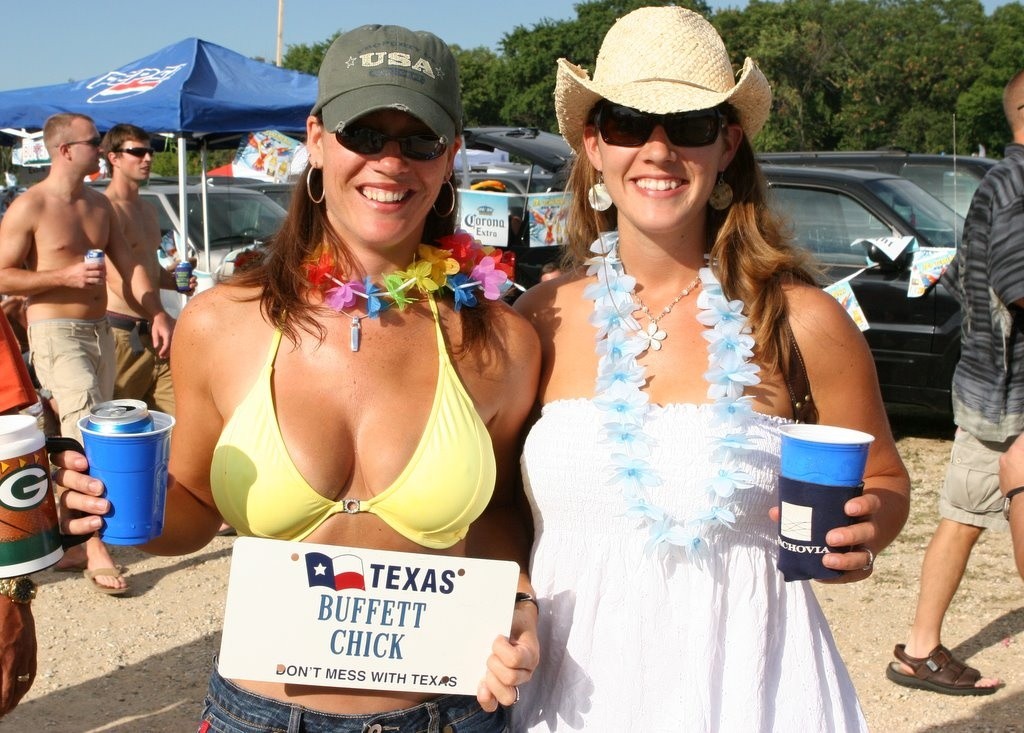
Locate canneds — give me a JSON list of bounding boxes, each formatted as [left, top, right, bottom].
[[176, 259, 192, 294], [86, 248, 103, 258], [87, 398, 153, 435]]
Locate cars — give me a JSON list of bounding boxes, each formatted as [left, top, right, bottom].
[[761, 164, 962, 417], [86, 177, 296, 323], [448, 125, 581, 310]]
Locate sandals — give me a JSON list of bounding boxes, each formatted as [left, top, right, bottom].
[[886, 642, 997, 696]]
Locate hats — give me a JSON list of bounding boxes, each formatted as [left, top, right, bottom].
[[555, 5, 772, 153], [309, 24, 462, 144]]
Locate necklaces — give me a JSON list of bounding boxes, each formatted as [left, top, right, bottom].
[[585, 231, 765, 554], [291, 232, 514, 352]]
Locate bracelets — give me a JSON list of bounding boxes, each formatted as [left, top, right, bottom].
[[517, 593, 539, 614], [0, 576, 38, 604]]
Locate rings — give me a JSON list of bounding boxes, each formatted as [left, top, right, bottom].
[[514, 687, 520, 704], [17, 674, 29, 681]]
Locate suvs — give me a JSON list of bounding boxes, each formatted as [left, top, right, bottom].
[[755, 152, 1002, 246]]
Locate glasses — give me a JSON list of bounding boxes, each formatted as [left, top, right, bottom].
[[336, 121, 447, 161], [114, 147, 155, 158], [61, 137, 101, 148], [598, 98, 720, 146]]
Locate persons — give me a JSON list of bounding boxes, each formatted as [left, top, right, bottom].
[[50, 23, 542, 733], [0, 114, 198, 595], [999, 432, 1024, 581], [511, 6, 911, 733], [0, 307, 30, 719], [886, 68, 1024, 696], [540, 262, 561, 281]]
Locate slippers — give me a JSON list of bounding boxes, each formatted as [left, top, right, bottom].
[[52, 558, 124, 573], [84, 566, 132, 595]]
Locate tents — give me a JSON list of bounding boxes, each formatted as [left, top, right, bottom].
[[0, 37, 321, 309]]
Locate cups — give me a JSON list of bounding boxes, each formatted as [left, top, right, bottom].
[[77, 411, 175, 545], [0, 414, 97, 580], [775, 424, 875, 583]]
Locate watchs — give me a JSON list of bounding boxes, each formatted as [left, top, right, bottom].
[[1003, 487, 1024, 520]]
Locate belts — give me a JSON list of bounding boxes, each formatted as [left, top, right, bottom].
[[108, 315, 153, 354]]
